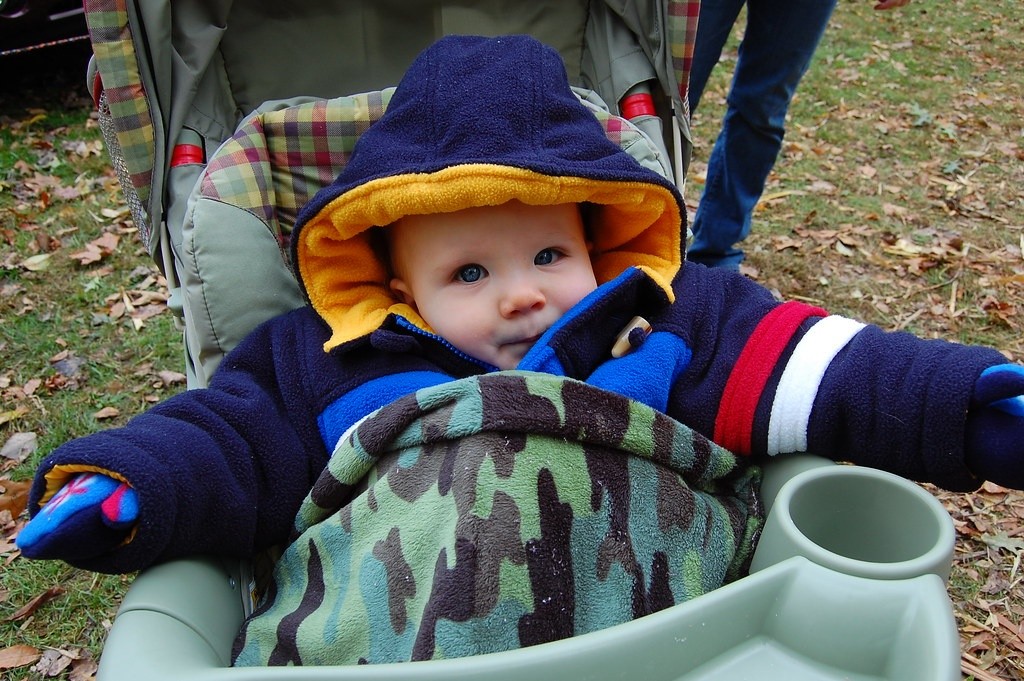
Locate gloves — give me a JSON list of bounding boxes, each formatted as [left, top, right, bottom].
[[14, 471, 141, 557], [966, 363, 1024, 490]]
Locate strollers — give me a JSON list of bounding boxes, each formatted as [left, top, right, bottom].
[[75, 0, 963, 681]]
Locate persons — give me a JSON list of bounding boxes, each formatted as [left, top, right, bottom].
[[684, 0, 911, 270], [16, 33, 1024, 668]]
